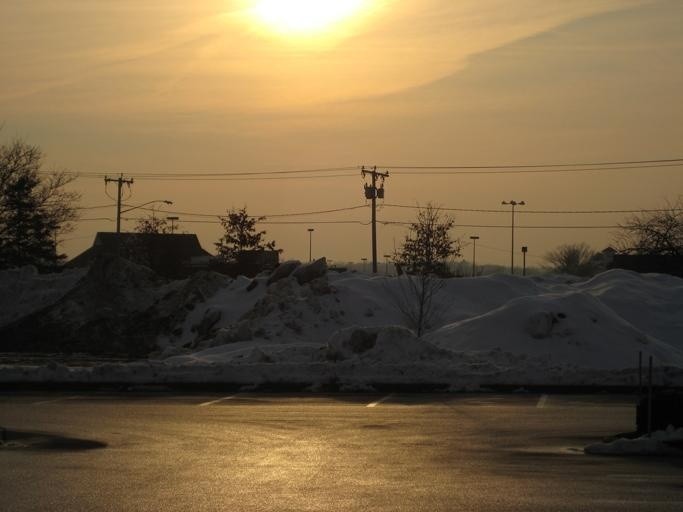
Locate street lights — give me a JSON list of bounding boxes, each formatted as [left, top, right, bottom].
[[306, 228, 315, 262], [501, 199, 526, 276], [470, 235, 479, 276], [383, 254, 391, 273], [116, 199, 172, 235], [359, 258, 367, 271]]
